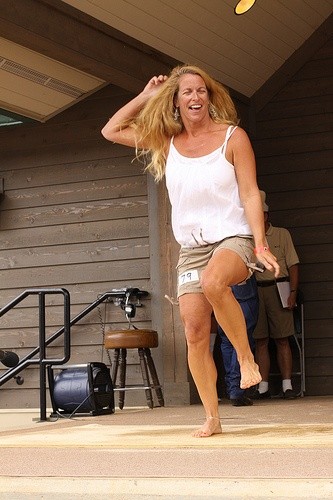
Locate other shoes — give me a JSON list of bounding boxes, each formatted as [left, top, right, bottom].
[[283, 389, 296, 400], [248, 389, 271, 400], [230, 397, 254, 406]]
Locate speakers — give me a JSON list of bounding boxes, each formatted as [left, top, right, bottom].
[[52, 367, 114, 413]]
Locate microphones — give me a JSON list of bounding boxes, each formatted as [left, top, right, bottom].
[[0, 350, 20, 368]]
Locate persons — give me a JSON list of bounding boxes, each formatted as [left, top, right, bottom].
[[217, 272, 260, 407], [253, 190, 300, 400], [100, 64, 280, 437]]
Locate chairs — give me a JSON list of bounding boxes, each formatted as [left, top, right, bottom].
[[252, 303, 305, 398]]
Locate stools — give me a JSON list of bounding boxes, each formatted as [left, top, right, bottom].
[[103, 329, 166, 411]]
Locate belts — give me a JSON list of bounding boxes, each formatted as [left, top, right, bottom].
[[256, 276, 291, 287]]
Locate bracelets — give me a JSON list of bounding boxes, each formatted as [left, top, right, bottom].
[[291, 290, 297, 292], [253, 247, 271, 253]]
